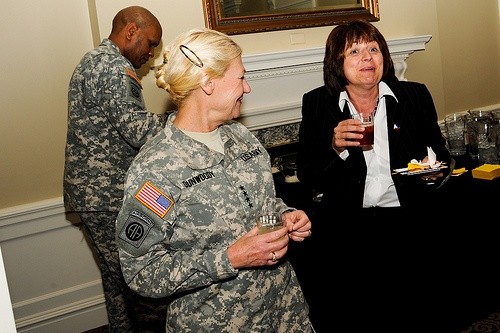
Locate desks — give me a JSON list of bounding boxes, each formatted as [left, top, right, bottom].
[[271, 139, 500, 333]]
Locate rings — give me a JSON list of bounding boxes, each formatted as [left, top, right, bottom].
[[309, 230, 311, 235], [272, 252, 276, 262]]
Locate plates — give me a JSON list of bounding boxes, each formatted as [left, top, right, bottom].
[[393, 165, 447, 175]]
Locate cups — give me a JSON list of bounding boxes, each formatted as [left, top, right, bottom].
[[351, 112, 375, 150], [255, 211, 287, 243], [443, 109, 500, 165]]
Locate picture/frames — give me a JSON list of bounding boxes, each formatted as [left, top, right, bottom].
[[202, 0, 381, 35]]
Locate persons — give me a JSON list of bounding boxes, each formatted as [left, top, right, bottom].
[[296, 21, 452, 225], [115, 29, 314, 333], [63, 6, 177, 332]]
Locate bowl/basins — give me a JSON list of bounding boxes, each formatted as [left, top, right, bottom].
[[274, 152, 300, 183]]
